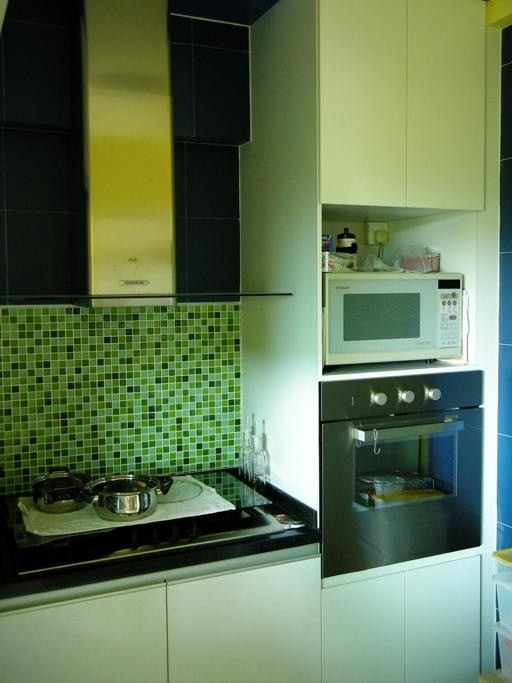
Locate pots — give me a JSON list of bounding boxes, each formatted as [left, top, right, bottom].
[[31, 466, 174, 522]]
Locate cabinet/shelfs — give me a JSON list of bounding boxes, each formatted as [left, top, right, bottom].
[[318, 0, 487, 209], [3, 557, 319, 680], [319, 553, 482, 682]]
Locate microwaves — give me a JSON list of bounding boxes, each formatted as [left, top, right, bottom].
[[322, 272, 467, 368]]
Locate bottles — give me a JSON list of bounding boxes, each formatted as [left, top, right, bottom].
[[336, 227, 356, 254]]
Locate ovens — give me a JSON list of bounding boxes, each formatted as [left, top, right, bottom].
[[319, 370, 484, 579]]
